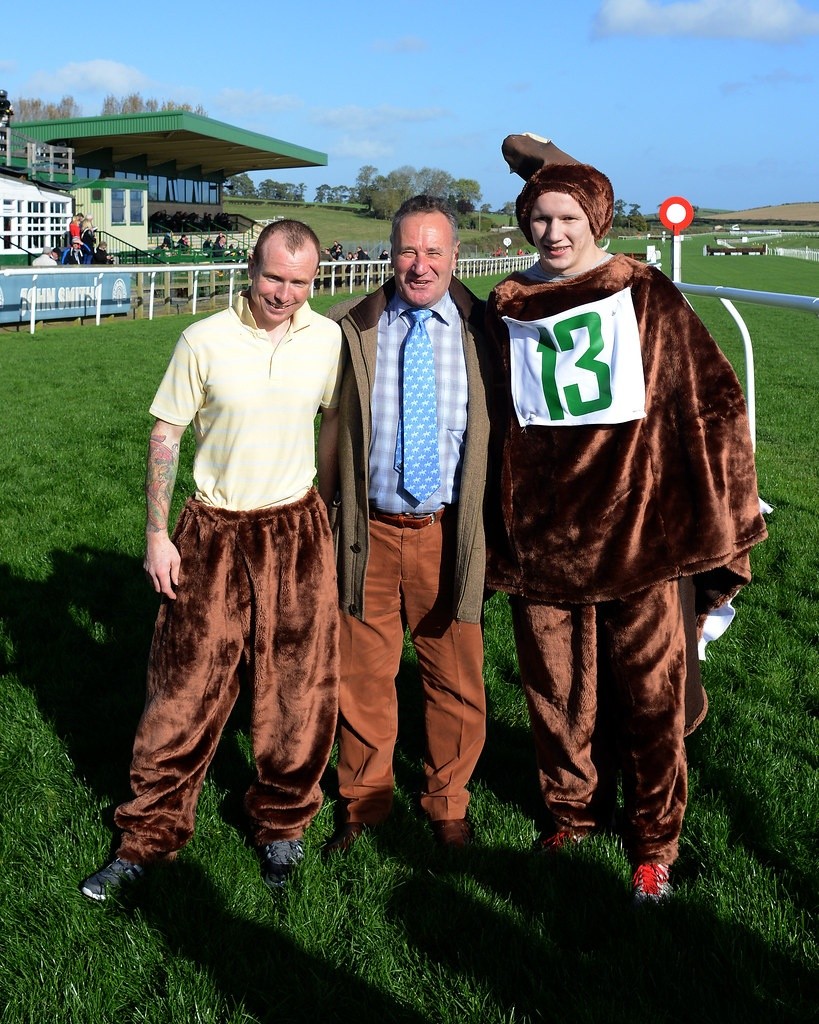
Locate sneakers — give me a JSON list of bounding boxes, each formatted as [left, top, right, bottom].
[[261, 839, 304, 889], [534, 827, 589, 869], [434, 820, 477, 873], [325, 823, 374, 864], [631, 862, 672, 907], [83, 858, 183, 902]]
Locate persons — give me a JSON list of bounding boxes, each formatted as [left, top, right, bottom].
[[0, 90, 13, 151], [329, 241, 371, 283], [322, 196, 493, 870], [203, 232, 253, 276], [484, 132, 767, 901], [81, 218, 343, 899], [517, 249, 530, 255], [32, 213, 114, 265], [155, 232, 189, 256], [380, 250, 388, 260], [495, 247, 501, 256]]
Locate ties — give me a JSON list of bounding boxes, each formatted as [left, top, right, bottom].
[[393, 310, 441, 504]]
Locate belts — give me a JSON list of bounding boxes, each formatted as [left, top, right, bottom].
[[370, 501, 459, 528]]
